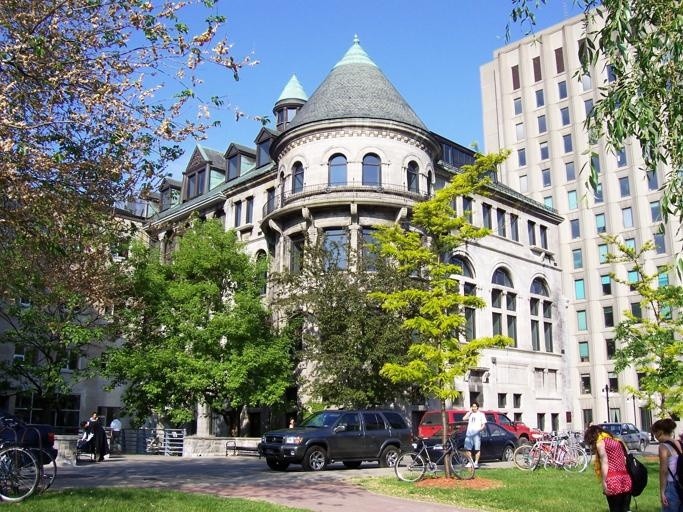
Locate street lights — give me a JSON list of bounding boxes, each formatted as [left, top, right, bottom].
[[626, 392, 643, 426], [601, 384, 612, 422]]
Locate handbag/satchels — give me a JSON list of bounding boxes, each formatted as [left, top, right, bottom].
[[619, 440, 647, 495]]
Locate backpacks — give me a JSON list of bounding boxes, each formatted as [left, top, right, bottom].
[[660, 439, 683, 498]]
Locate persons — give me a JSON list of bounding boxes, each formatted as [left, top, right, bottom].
[[124, 412, 133, 426], [110, 416, 122, 454], [585, 425, 633, 512], [90, 412, 98, 423], [289, 417, 296, 429], [81, 420, 109, 462], [108, 413, 120, 427], [340, 415, 355, 429], [461, 400, 487, 469], [99, 411, 106, 423], [650, 418, 682, 512]]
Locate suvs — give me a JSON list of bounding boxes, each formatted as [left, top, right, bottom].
[[254, 406, 414, 473]]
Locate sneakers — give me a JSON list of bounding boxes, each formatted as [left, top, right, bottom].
[[464, 462, 480, 469]]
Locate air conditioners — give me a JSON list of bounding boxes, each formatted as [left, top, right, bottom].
[[19, 297, 31, 308], [13, 354, 25, 367]]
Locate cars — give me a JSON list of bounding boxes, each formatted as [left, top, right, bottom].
[[418, 409, 653, 466]]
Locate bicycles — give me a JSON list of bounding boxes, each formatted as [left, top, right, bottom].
[[0, 414, 60, 505], [512, 430, 593, 473], [391, 431, 479, 482]]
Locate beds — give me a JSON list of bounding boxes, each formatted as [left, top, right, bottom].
[[226, 441, 262, 459]]
